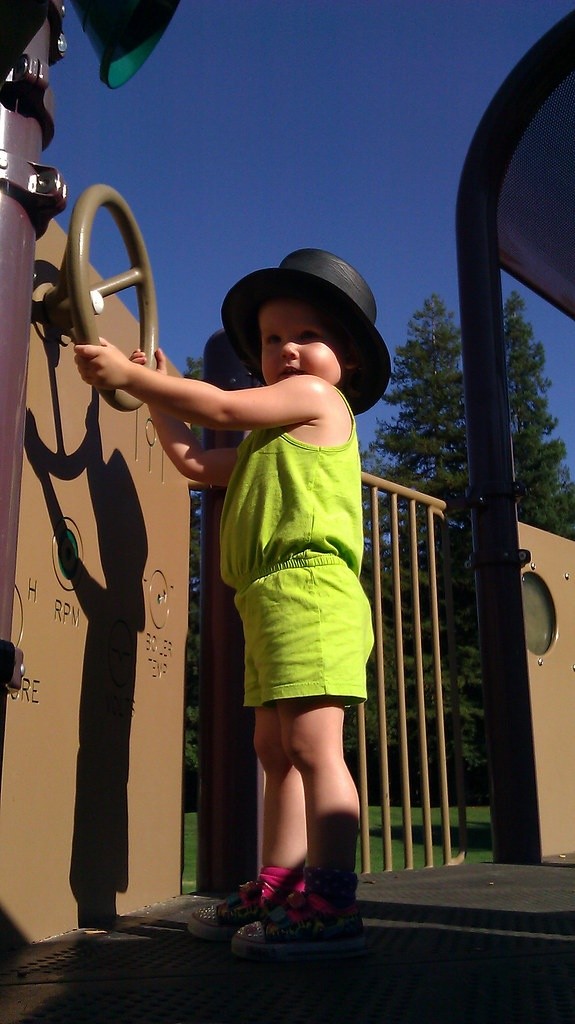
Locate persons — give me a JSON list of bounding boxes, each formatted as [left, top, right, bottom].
[[73, 248, 391, 959]]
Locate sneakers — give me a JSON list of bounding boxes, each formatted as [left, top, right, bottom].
[[231, 891, 368, 964], [187, 880, 285, 942]]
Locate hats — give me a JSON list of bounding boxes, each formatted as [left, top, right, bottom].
[[221, 248, 391, 416]]
